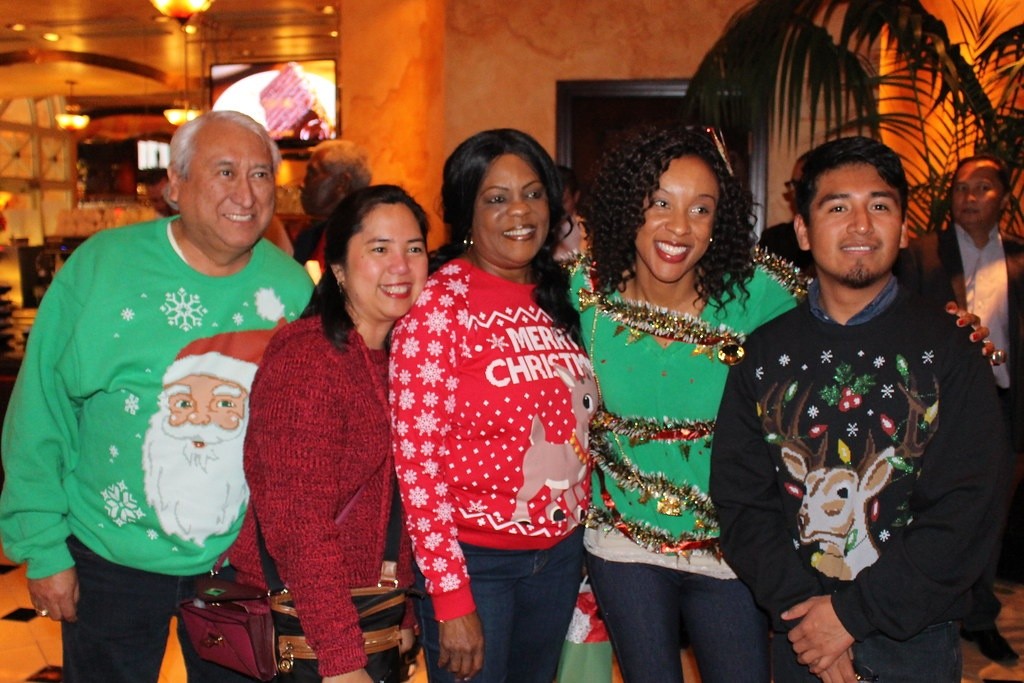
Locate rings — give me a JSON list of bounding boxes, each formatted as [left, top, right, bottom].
[[36, 608, 48, 616]]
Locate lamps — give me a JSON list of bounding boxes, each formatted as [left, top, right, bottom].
[[150, 0, 215, 127], [55, 80, 90, 132]]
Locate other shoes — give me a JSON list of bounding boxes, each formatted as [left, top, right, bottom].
[[959, 622, 1020, 664]]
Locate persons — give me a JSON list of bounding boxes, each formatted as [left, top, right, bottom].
[[386, 126, 597, 683], [211, 184, 428, 683], [709, 137, 1006, 682], [584, 123, 1005, 683], [906, 160, 1024, 662], [294, 139, 369, 286], [760, 150, 816, 275], [0, 111, 315, 683], [558, 167, 594, 308]]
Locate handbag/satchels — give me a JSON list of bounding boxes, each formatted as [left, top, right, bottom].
[[179, 575, 278, 682], [268, 584, 428, 683]]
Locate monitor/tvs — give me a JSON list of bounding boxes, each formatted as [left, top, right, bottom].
[[210, 58, 339, 149]]
